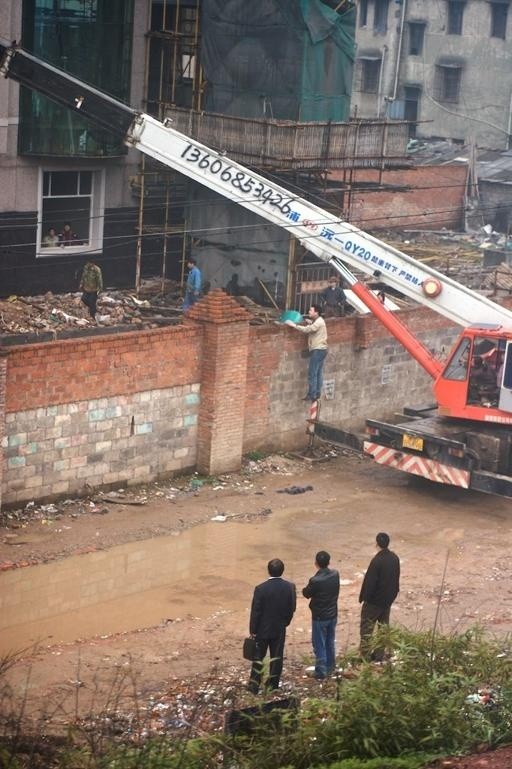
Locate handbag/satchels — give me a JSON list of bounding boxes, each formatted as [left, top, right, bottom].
[[243, 637, 260, 662]]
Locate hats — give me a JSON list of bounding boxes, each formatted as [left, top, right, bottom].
[[327, 276, 341, 283]]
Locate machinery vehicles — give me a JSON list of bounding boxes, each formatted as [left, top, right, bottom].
[[0, 38, 512, 504]]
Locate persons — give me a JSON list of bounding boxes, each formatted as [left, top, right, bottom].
[[79, 259, 103, 321], [302, 550, 339, 680], [59, 221, 83, 245], [183, 256, 202, 311], [359, 531, 400, 661], [42, 224, 61, 248], [320, 276, 346, 319], [461, 355, 497, 407], [283, 303, 328, 402], [244, 558, 297, 694]]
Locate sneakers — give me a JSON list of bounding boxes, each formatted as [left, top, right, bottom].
[[244, 682, 278, 696], [300, 395, 321, 403]]
